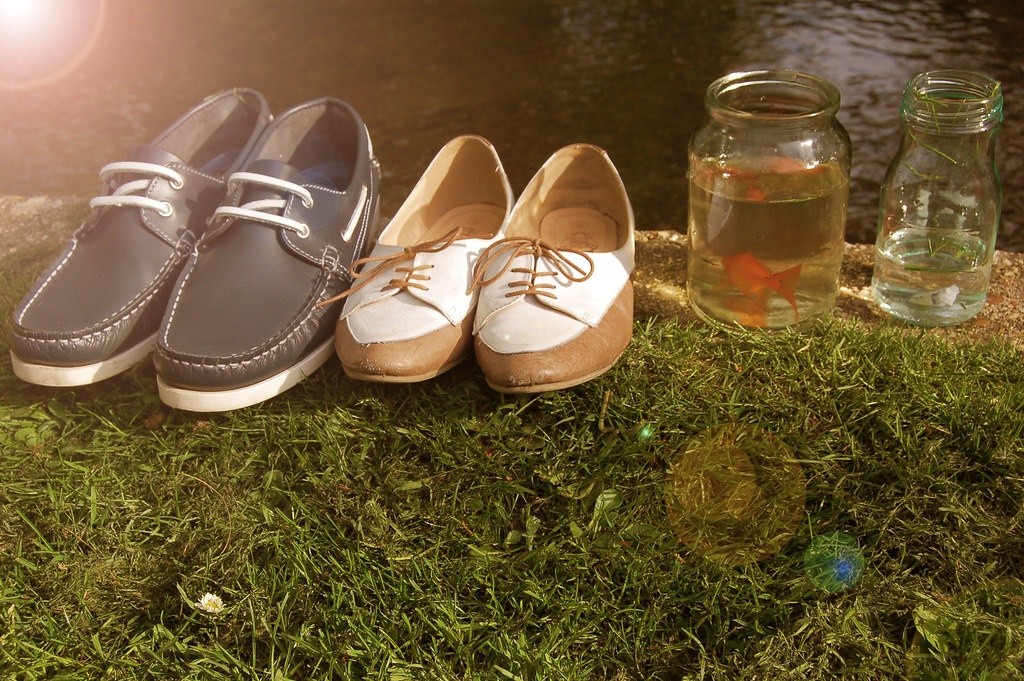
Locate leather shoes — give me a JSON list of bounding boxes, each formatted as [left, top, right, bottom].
[[9, 86, 382, 413], [333, 134, 635, 394]]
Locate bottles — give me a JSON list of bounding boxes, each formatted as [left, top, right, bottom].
[[866, 69, 1004, 328], [687, 69, 852, 335]]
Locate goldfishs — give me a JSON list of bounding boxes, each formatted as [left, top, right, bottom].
[[700, 150, 833, 202], [720, 253, 802, 312]]
